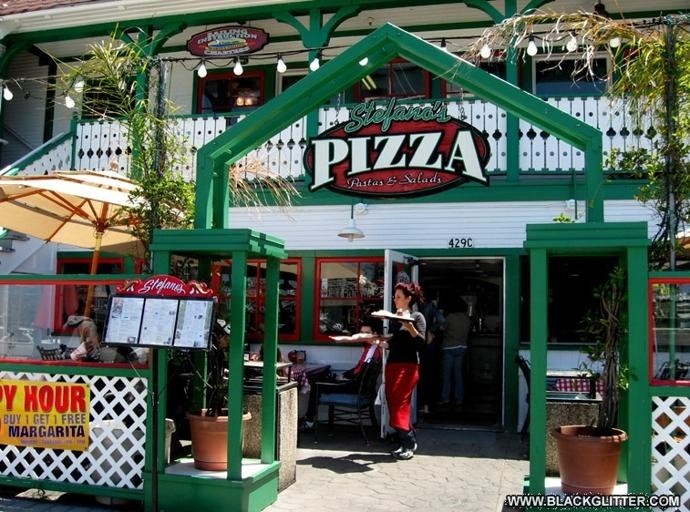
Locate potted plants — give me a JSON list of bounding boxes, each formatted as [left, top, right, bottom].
[[185, 349, 252, 472], [548, 264, 639, 497]]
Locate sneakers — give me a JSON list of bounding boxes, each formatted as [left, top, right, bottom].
[[391, 443, 418, 460]]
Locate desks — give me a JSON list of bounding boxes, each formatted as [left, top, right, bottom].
[[546, 370, 605, 405], [285, 363, 331, 394]]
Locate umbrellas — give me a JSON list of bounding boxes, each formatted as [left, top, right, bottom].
[[1, 159, 188, 347]]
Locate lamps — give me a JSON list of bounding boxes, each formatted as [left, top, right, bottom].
[[236, 92, 257, 106], [337, 195, 364, 242]]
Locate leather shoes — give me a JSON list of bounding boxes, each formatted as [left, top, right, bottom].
[[298, 417, 315, 431]]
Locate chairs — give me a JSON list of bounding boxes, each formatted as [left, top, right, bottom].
[[514, 354, 557, 445], [314, 358, 382, 444], [36, 344, 64, 360], [653, 358, 690, 456]]
